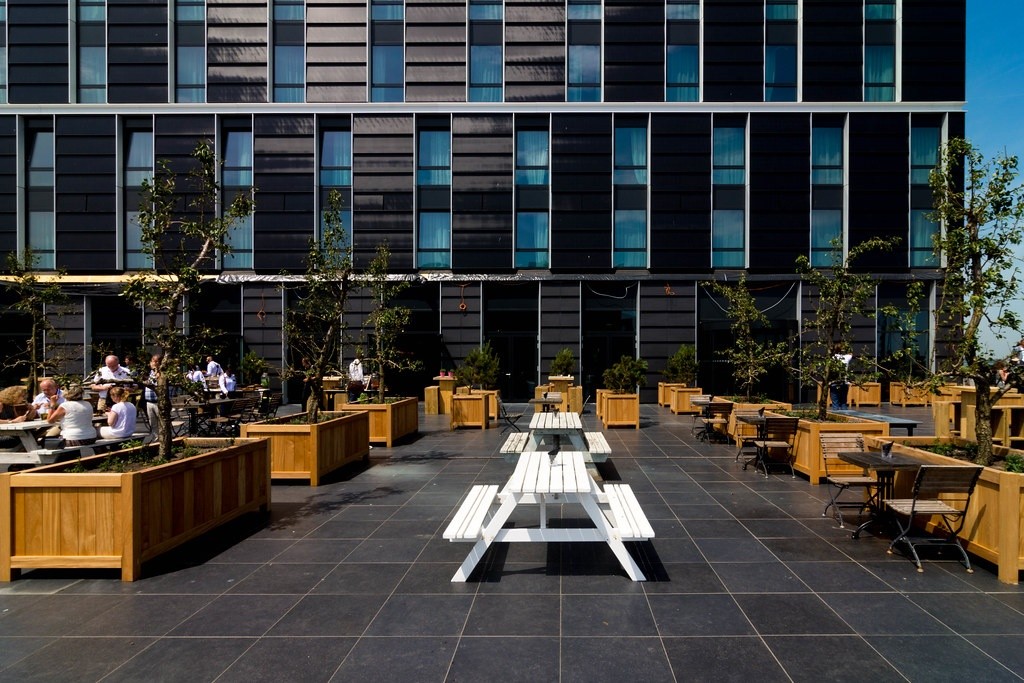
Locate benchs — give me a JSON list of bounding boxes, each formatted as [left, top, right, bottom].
[[30, 432, 150, 468], [442, 432, 656, 541], [829, 410, 926, 436]]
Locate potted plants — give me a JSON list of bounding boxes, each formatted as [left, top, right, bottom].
[[862, 134, 1024, 587], [769, 234, 903, 486], [0, 137, 269, 582], [237, 187, 422, 487], [441, 272, 1024, 443]]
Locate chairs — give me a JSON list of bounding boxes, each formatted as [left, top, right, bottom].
[[541, 392, 562, 412], [560, 395, 590, 439], [138, 385, 283, 445], [494, 395, 523, 435], [689, 395, 984, 573]]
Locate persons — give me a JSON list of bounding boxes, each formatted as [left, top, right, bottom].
[[185, 354, 224, 392], [90, 355, 136, 412], [261, 371, 269, 388], [991, 339, 1024, 395], [346, 353, 365, 404], [218, 364, 237, 428], [0, 379, 137, 447], [824, 341, 854, 410], [301, 357, 327, 412], [142, 353, 164, 442]]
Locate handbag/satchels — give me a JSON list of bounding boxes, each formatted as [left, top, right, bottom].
[[219, 393, 227, 399]]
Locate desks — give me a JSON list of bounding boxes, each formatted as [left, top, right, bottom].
[[433, 376, 463, 414], [933, 386, 1018, 424], [692, 401, 937, 540], [0, 377, 274, 468], [323, 389, 389, 412], [959, 390, 1024, 444], [450, 392, 647, 583]]
